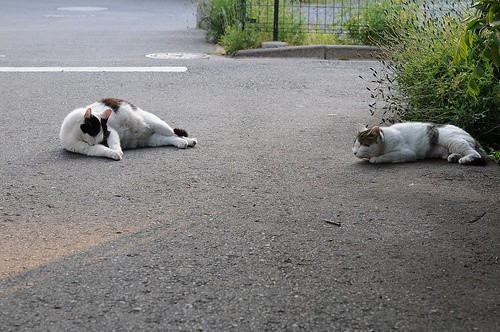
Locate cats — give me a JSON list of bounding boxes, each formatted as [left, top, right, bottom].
[[353, 121, 487, 164], [60, 97, 198, 161]]
